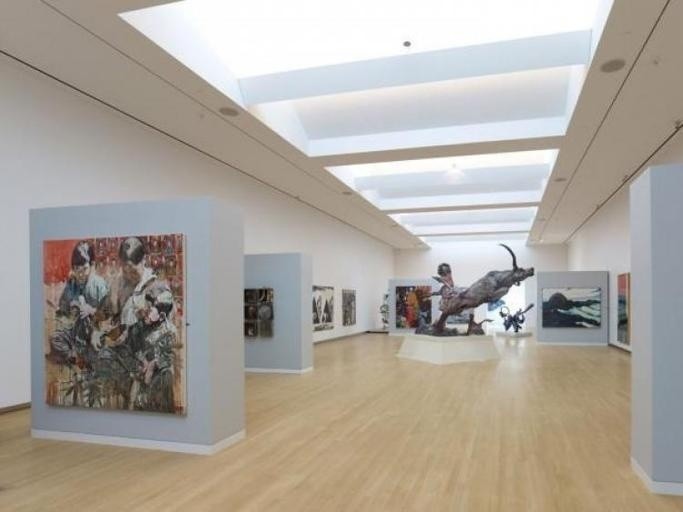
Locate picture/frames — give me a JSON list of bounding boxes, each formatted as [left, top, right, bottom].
[[617, 272, 630, 346]]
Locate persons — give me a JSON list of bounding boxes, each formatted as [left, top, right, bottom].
[[49, 238, 179, 412]]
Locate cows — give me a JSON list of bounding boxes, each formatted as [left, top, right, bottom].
[[412, 243, 535, 330]]
